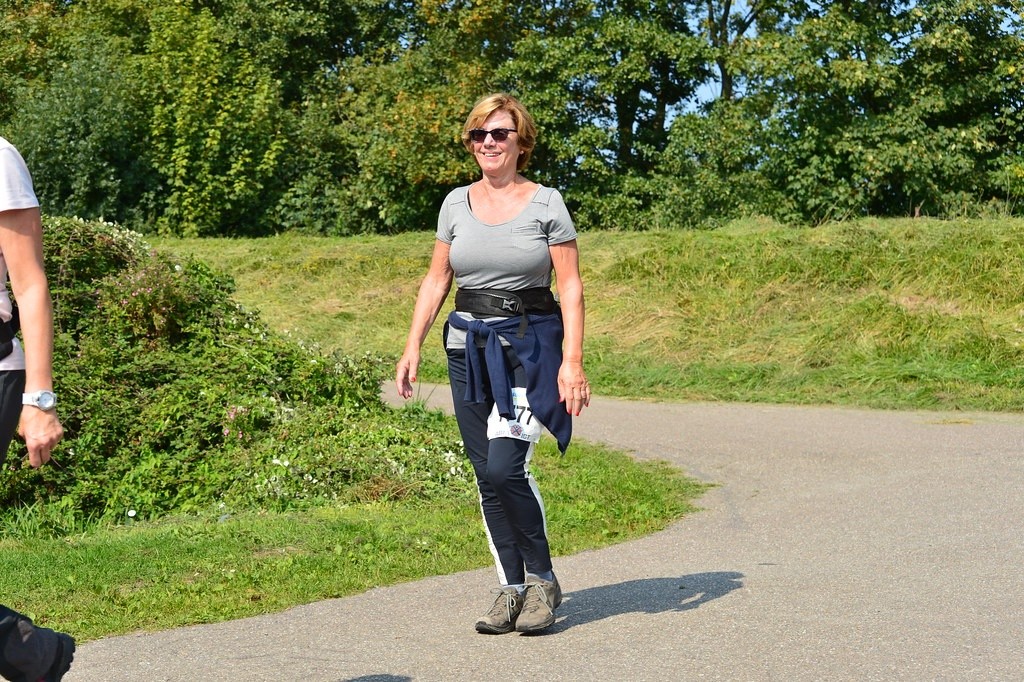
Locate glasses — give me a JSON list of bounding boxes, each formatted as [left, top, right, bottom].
[[470, 127, 518, 142]]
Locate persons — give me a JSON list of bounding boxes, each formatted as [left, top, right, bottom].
[[0, 134, 75, 682], [395, 96, 591, 635]]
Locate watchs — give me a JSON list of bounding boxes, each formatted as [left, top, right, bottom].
[[22, 389, 57, 411]]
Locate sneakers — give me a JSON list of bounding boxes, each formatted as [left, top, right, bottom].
[[12, 628, 75, 682], [516, 569, 564, 632], [475, 587, 525, 634]]
